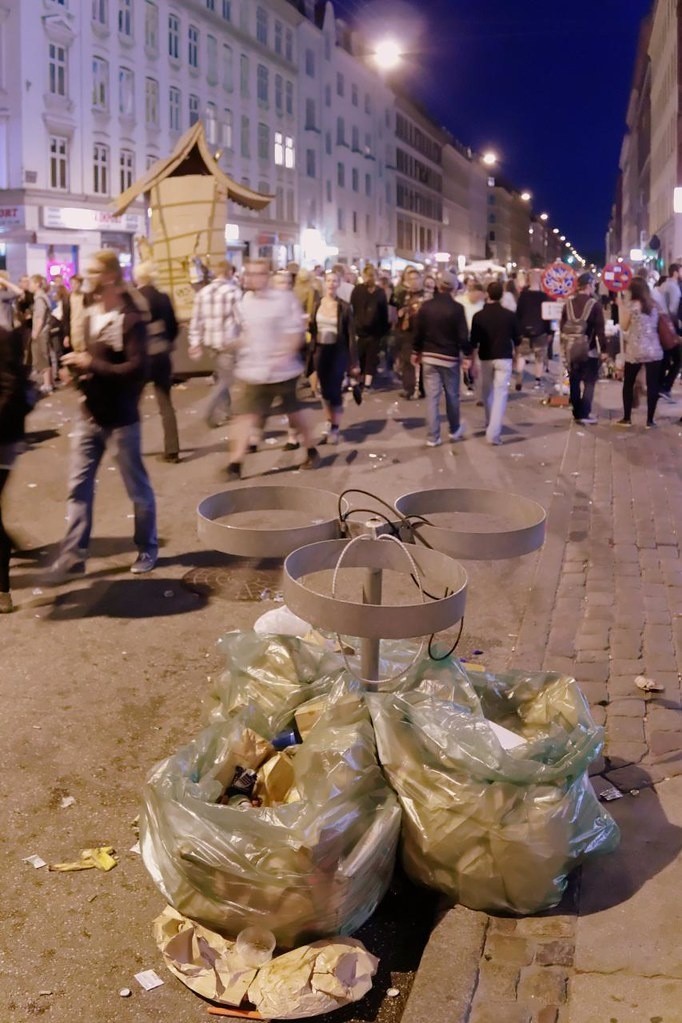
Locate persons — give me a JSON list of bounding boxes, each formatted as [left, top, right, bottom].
[[617, 277, 664, 429], [560, 272, 609, 426], [45, 252, 160, 582], [304, 272, 361, 445], [409, 272, 471, 447], [469, 281, 522, 446], [0, 251, 682, 616]]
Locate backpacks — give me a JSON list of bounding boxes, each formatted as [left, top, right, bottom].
[[561, 299, 597, 364]]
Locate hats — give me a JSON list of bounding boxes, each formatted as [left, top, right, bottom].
[[577, 272, 597, 288], [438, 271, 458, 288]]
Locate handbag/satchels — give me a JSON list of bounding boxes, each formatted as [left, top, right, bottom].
[[353, 373, 362, 405], [655, 303, 679, 349]]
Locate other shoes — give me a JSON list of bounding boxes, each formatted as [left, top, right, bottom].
[[219, 462, 241, 479], [23, 358, 62, 392], [299, 446, 320, 469], [246, 445, 258, 455], [0, 592, 13, 614], [204, 418, 218, 428], [281, 442, 300, 451], [427, 437, 441, 447], [130, 552, 158, 573], [468, 364, 676, 430], [320, 420, 339, 445], [155, 451, 180, 463], [39, 557, 86, 585], [448, 423, 463, 440], [399, 392, 410, 400]]
